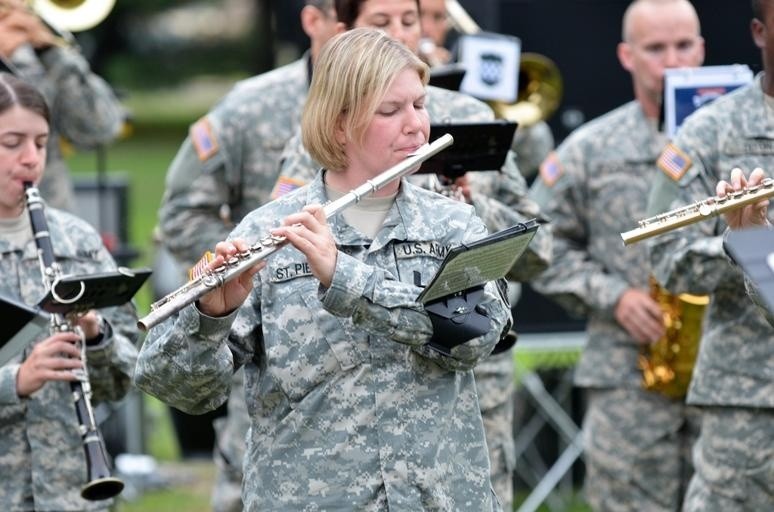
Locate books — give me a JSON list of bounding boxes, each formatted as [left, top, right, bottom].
[[449, 28, 523, 107], [659, 60, 758, 146]]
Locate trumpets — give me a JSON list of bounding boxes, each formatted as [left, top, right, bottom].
[[28, 0, 115, 36], [420, 0, 563, 127]]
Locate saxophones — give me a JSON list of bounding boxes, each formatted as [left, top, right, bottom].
[[638, 268, 710, 402]]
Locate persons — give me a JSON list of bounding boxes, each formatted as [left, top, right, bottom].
[[133, 24, 516, 512], [0, 66, 148, 511], [273, 1, 538, 511], [712, 161, 774, 316], [1, 1, 131, 215], [646, 0, 774, 512], [157, 0, 350, 512], [418, 0, 553, 189], [516, 0, 757, 512]]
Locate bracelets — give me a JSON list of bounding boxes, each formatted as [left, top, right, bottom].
[[80, 316, 107, 348]]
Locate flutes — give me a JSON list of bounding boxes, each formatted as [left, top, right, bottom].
[[621, 180, 774, 248], [136, 132, 454, 334]]
